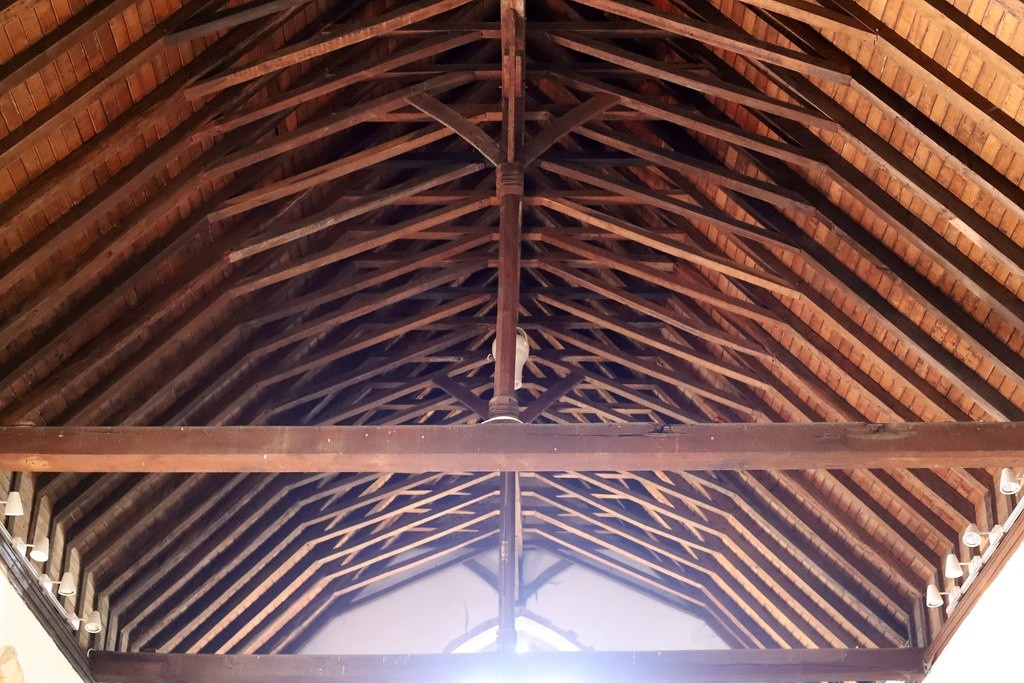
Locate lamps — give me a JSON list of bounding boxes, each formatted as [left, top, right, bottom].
[[944, 553, 984, 579], [999, 466, 1024, 496], [0, 491, 24, 516], [67, 611, 102, 634], [12, 535, 50, 564], [962, 523, 1004, 548], [38, 572, 77, 597], [926, 584, 962, 609]]
